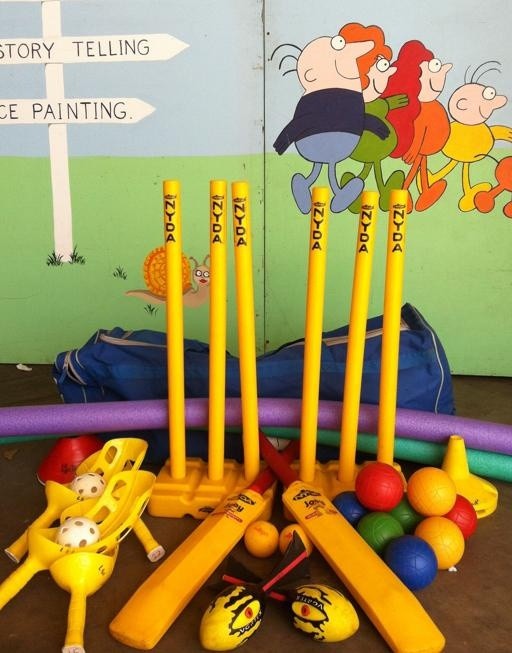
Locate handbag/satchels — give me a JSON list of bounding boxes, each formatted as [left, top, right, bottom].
[[51, 302, 457, 463]]
